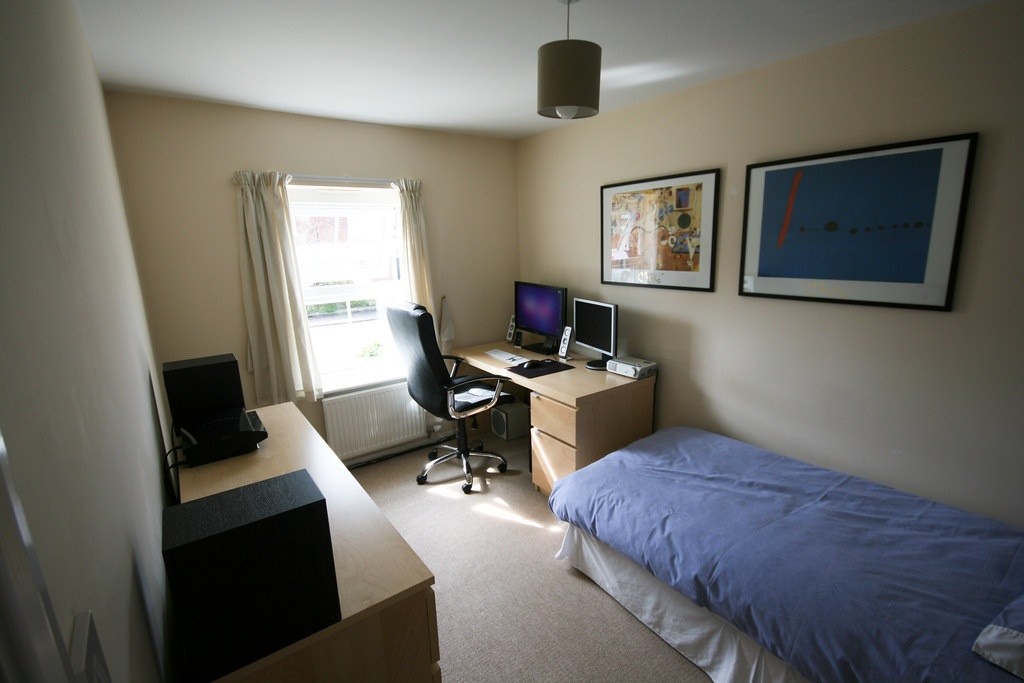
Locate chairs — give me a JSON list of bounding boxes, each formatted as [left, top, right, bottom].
[[386, 300, 516, 495]]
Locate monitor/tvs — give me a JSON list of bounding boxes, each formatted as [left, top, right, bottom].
[[514, 281, 568, 355], [574, 298, 618, 370]]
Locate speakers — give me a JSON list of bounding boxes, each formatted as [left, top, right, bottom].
[[492, 401, 529, 441], [559, 326, 572, 361], [506, 315, 515, 343]]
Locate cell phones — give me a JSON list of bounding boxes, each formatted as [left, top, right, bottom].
[[515, 332, 522, 346]]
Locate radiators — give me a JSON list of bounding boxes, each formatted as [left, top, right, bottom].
[[320, 382, 442, 462]]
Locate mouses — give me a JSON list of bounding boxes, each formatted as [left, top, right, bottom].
[[524, 360, 542, 369]]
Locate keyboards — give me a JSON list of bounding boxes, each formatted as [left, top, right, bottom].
[[485, 348, 529, 366]]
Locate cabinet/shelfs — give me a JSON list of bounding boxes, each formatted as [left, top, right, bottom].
[[451, 340, 657, 496], [176, 401, 442, 683]]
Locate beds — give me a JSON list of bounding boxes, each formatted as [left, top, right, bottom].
[[548, 426, 1024, 683]]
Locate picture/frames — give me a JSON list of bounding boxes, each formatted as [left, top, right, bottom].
[[737, 130, 979, 314], [600, 166, 721, 291]]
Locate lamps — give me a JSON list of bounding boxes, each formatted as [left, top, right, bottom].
[[536, 0, 603, 121]]
